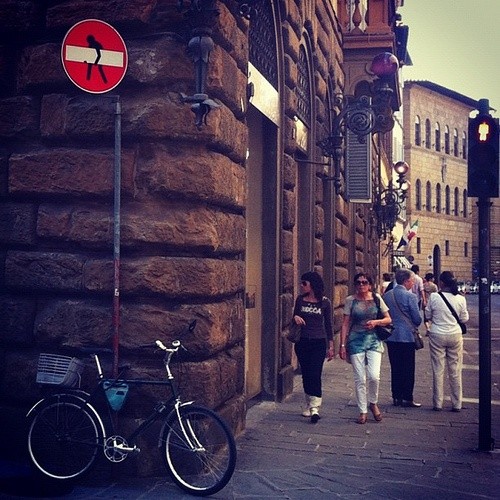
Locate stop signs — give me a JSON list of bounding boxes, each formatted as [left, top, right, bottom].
[[60, 19, 129, 95]]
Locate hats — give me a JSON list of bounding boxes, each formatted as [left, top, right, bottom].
[[424, 273, 435, 279]]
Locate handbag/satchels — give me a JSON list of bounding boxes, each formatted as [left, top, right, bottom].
[[414, 327, 424, 350], [438, 291, 467, 334], [372, 292, 394, 340], [281, 323, 302, 343]]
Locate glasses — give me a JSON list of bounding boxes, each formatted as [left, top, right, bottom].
[[301, 281, 310, 286], [354, 279, 370, 286]]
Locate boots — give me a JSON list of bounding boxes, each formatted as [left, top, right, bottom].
[[303, 394, 311, 416], [305, 394, 322, 423]]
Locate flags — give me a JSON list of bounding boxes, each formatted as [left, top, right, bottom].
[[397, 220, 418, 250]]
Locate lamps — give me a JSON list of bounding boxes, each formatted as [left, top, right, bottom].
[[372, 161, 411, 241], [294, 52, 399, 196]]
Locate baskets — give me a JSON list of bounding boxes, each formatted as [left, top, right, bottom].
[[36, 353, 85, 388]]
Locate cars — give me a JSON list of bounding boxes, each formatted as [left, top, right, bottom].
[[457, 281, 500, 295]]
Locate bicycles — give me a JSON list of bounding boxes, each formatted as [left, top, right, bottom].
[[23, 338, 238, 498]]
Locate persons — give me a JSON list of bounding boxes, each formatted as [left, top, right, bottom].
[[383, 269, 423, 408], [425, 271, 469, 413], [382, 265, 439, 337], [340, 272, 392, 423], [293, 272, 334, 424]]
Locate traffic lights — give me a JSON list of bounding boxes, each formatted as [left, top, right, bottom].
[[467, 108, 498, 198]]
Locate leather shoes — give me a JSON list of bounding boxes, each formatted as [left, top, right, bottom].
[[400, 400, 422, 407], [393, 398, 400, 405]]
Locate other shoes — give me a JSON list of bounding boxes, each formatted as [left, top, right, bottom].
[[433, 407, 442, 411], [369, 403, 382, 421], [358, 413, 368, 424], [454, 409, 462, 412], [426, 331, 429, 337]]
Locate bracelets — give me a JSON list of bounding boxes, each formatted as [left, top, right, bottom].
[[341, 344, 344, 348]]
